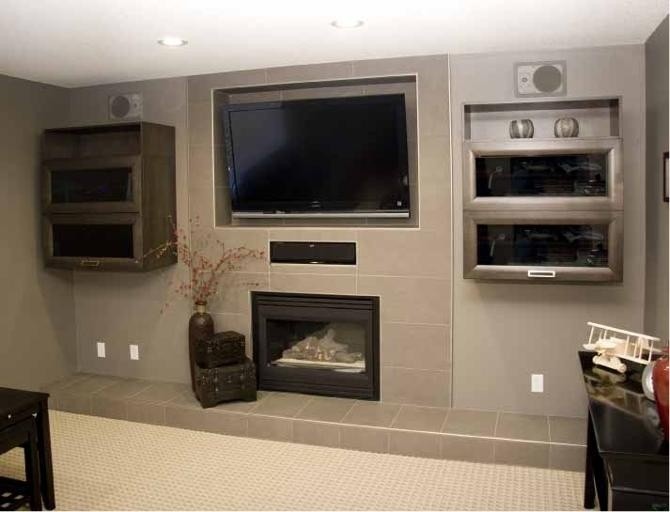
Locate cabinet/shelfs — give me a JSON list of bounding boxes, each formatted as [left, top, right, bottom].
[[461, 94, 625, 284], [38, 119, 177, 274]]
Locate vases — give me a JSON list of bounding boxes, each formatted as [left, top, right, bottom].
[[189, 302, 214, 392]]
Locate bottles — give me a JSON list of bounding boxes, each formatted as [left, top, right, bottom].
[[653, 346, 669, 446]]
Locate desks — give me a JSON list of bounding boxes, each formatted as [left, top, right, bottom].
[[577, 349, 669, 511]]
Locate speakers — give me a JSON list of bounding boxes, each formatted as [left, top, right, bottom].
[[515, 60, 569, 97], [269, 240, 356, 263], [107, 90, 142, 118]]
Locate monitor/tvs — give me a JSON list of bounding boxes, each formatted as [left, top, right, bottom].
[[219, 92, 410, 219]]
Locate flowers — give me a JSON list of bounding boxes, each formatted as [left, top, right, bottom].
[[133, 209, 266, 316]]
